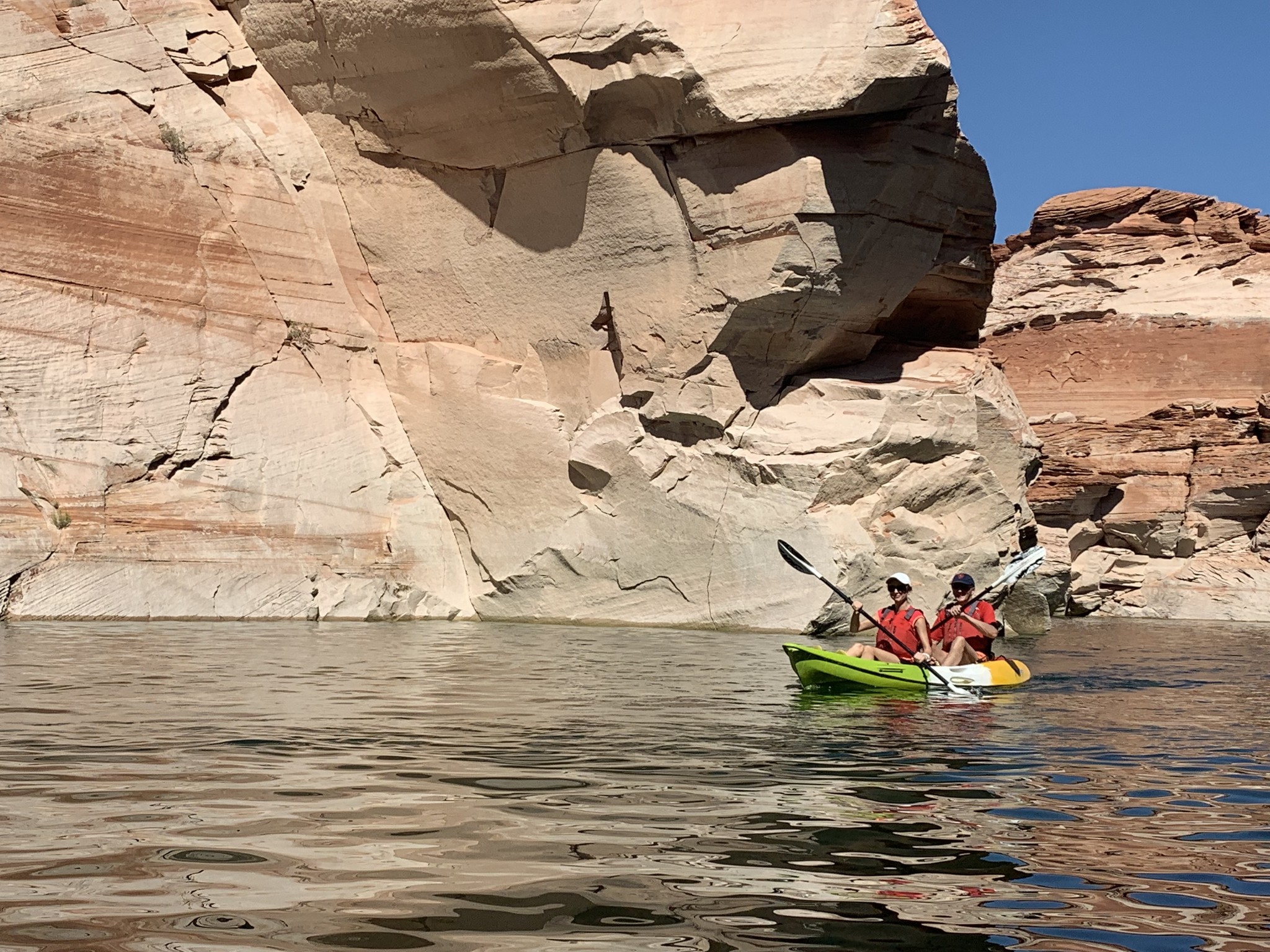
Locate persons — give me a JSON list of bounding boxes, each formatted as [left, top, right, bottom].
[[815, 572, 932, 664], [930, 573, 998, 666]]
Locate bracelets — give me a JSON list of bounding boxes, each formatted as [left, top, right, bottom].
[[922, 653, 930, 659]]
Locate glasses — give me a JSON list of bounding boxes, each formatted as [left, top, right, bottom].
[[887, 584, 908, 592], [952, 584, 969, 590]]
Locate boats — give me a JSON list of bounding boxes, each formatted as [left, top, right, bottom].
[[782, 642, 1032, 696]]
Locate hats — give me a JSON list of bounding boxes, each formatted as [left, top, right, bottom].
[[886, 572, 912, 588], [949, 573, 975, 586]]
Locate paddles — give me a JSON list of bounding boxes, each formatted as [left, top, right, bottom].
[[930, 545, 1047, 632], [777, 539, 970, 695]]
